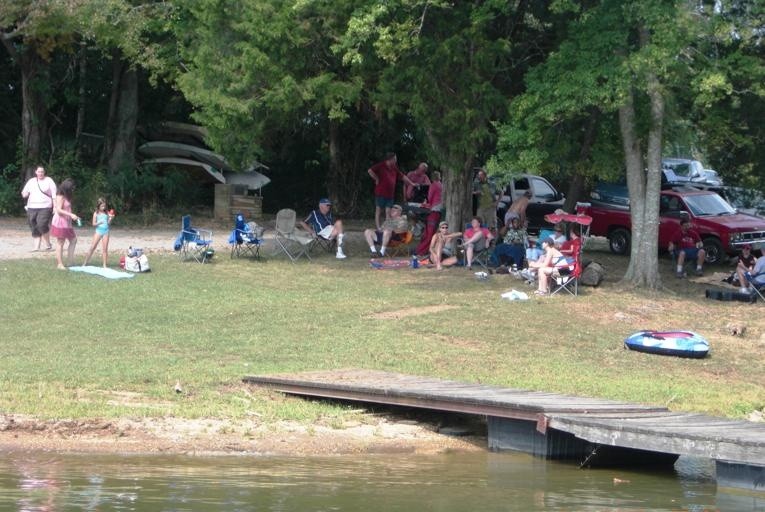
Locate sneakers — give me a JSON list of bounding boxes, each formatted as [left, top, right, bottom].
[[676, 270, 683, 277], [339, 235, 344, 244], [696, 267, 703, 276], [336, 252, 347, 259], [465, 265, 471, 270], [522, 273, 534, 282], [742, 287, 747, 293], [370, 251, 384, 257], [58, 262, 65, 270], [534, 290, 549, 295], [457, 244, 465, 250], [512, 265, 518, 273], [427, 264, 432, 267]]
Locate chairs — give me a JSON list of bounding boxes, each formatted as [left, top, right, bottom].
[[173, 208, 336, 264], [383, 229, 413, 261], [464, 223, 580, 297]]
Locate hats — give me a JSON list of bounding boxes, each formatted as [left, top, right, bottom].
[[431, 171, 441, 180], [742, 244, 751, 249], [320, 199, 331, 204], [541, 238, 554, 245], [391, 205, 402, 211]]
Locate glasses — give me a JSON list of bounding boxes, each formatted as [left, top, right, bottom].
[[441, 226, 448, 229]]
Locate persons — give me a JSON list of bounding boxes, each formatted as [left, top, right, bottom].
[[667, 219, 706, 279], [729, 242, 757, 285], [363, 151, 582, 296], [300, 198, 348, 259], [81, 197, 117, 269], [49, 177, 78, 271], [736, 244, 765, 294], [21, 164, 57, 253]]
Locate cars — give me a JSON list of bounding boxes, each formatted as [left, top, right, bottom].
[[496, 175, 567, 233], [588, 180, 631, 210]]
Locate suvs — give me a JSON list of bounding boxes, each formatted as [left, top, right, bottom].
[[660, 156, 765, 217]]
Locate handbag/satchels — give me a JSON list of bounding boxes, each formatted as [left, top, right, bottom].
[[125, 254, 149, 272]]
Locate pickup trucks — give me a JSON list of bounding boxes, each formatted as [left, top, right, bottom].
[[575, 185, 765, 266]]
